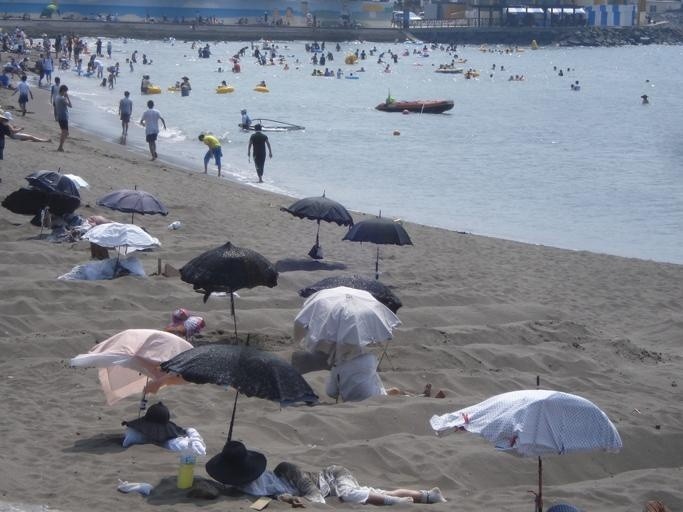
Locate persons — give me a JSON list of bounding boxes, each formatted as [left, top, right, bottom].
[[641, 95, 648, 104], [306, 12, 571, 80], [237, 462, 448, 508], [82, 221, 162, 279], [0, 6, 289, 183], [571, 80, 580, 91]]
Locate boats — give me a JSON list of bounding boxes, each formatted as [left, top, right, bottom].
[[373, 97, 452, 114], [478, 48, 488, 51], [435, 67, 463, 73], [456, 59, 466, 63], [516, 48, 524, 52], [311, 48, 322, 53], [465, 71, 479, 79]]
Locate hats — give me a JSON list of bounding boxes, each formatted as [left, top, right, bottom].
[[121, 401, 187, 442], [205, 442, 267, 485], [0, 112, 13, 120], [120, 257, 145, 276]]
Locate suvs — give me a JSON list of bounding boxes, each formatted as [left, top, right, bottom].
[[417, 12, 425, 21]]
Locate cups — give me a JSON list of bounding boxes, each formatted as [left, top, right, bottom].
[[175, 463, 196, 489]]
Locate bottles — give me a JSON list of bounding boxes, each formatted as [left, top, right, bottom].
[[167, 220, 182, 231]]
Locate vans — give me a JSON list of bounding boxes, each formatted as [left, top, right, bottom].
[[392, 11, 422, 25]]
